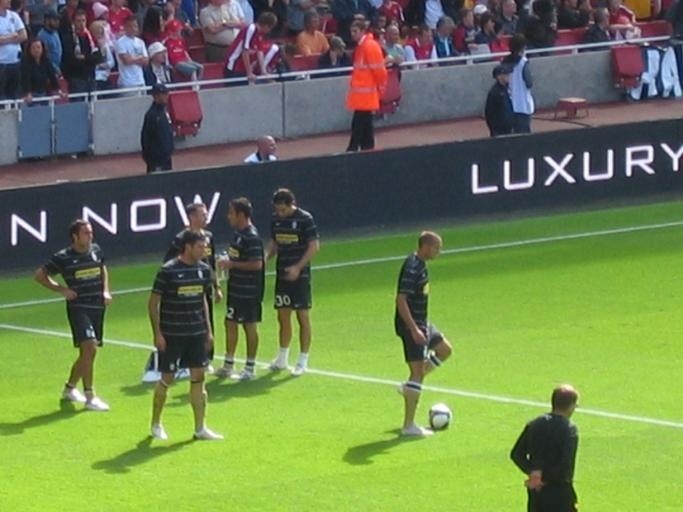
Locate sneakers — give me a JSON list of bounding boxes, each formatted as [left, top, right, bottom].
[[212, 367, 236, 378], [193, 427, 224, 441], [141, 371, 162, 382], [63, 388, 88, 402], [151, 424, 167, 441], [230, 368, 256, 382], [262, 358, 288, 371], [291, 362, 308, 376], [85, 396, 109, 411], [401, 423, 433, 436]]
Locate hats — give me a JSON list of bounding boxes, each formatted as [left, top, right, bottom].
[[473, 4, 490, 15], [166, 20, 184, 33], [92, 2, 109, 19], [493, 65, 512, 77], [44, 8, 63, 19], [147, 41, 167, 57]]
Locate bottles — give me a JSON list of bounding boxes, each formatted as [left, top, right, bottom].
[[219, 250, 229, 278]]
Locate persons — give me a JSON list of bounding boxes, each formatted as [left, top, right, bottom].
[[508, 382, 582, 512], [393, 228, 455, 437], [1, 2, 642, 109], [345, 19, 388, 153], [213, 195, 268, 383], [484, 32, 534, 136], [140, 84, 175, 175], [243, 133, 278, 163], [141, 202, 226, 386], [147, 225, 227, 442], [32, 218, 115, 412], [263, 186, 321, 378]]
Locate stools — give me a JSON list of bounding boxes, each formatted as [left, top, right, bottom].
[[551, 96, 590, 121]]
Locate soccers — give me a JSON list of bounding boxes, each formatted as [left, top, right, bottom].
[[429, 403, 450, 430]]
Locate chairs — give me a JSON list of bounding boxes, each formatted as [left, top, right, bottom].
[[53, 3, 681, 102]]
